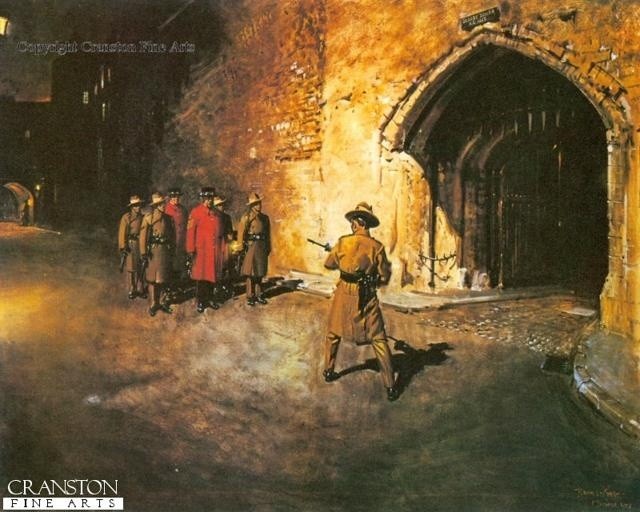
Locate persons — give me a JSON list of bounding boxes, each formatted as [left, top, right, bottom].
[[20, 199, 31, 226], [322, 203, 401, 400], [116, 185, 274, 316]]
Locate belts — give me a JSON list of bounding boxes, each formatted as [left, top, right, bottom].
[[151, 236, 168, 243], [127, 234, 139, 240], [339, 270, 361, 285], [247, 233, 265, 241]]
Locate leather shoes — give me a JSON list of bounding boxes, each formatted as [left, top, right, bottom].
[[128, 290, 147, 299], [149, 307, 156, 316], [197, 302, 203, 313], [161, 305, 172, 312], [386, 387, 398, 400], [323, 367, 334, 382], [207, 300, 219, 310], [247, 299, 254, 305], [255, 296, 266, 303]]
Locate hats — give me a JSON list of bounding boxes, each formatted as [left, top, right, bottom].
[[246, 191, 266, 206], [150, 192, 168, 207], [169, 188, 185, 196], [198, 187, 215, 197], [128, 194, 146, 208], [344, 202, 380, 229], [214, 195, 228, 206]]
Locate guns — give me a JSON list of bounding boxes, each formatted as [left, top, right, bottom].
[[237, 252, 243, 273], [119, 249, 128, 272], [140, 224, 153, 281], [185, 252, 193, 278], [308, 239, 332, 251]]
